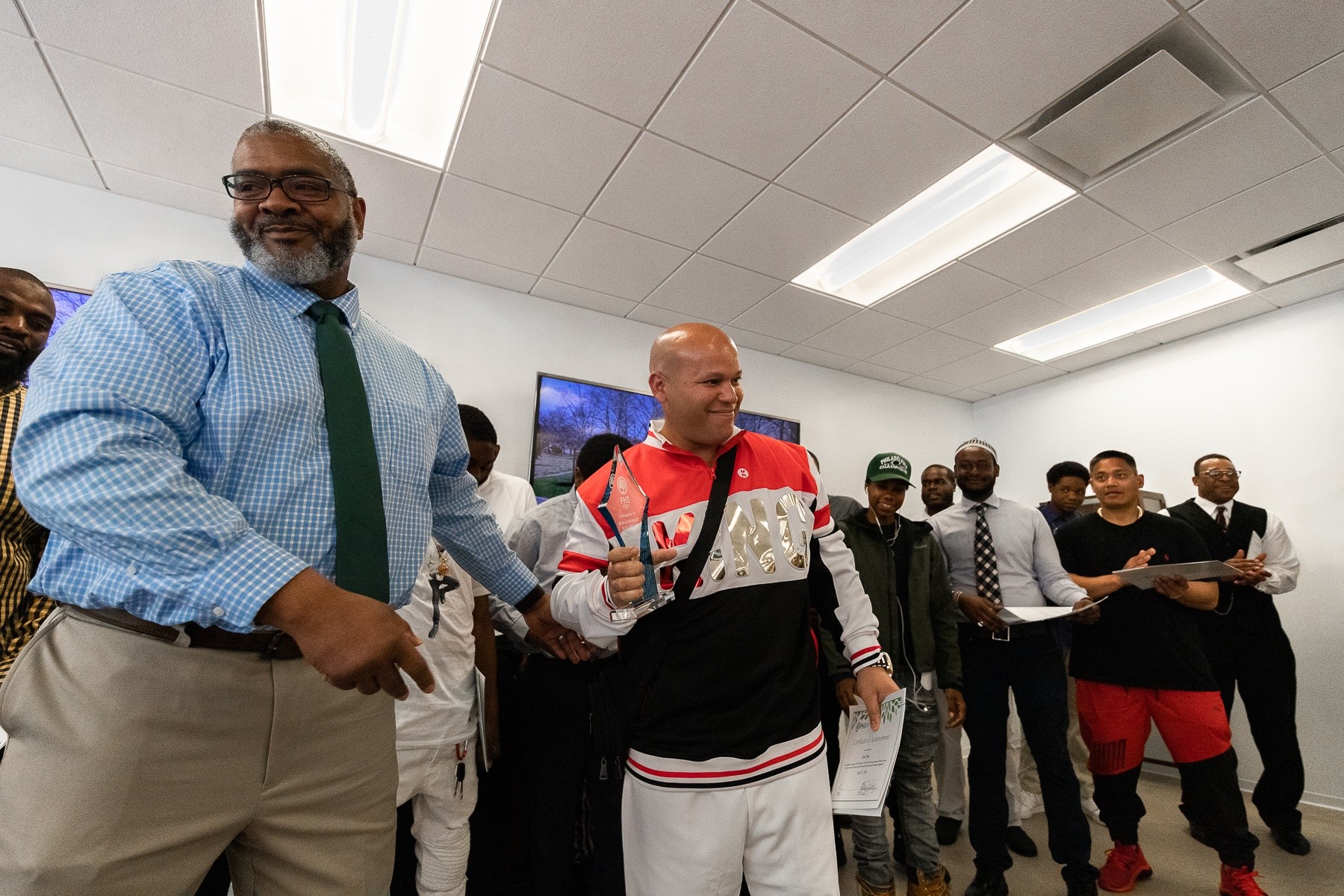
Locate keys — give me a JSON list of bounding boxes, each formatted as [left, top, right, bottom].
[[453, 764, 459, 797], [457, 763, 466, 799]]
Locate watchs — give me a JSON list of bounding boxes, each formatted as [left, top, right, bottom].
[[872, 651, 894, 678]]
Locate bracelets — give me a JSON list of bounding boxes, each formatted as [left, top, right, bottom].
[[513, 582, 545, 614], [950, 590, 963, 604]]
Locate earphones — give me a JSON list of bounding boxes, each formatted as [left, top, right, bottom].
[[866, 487, 868, 494]]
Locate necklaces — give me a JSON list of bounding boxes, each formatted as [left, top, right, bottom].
[[433, 535, 449, 575]]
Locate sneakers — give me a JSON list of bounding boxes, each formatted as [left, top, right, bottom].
[[1019, 790, 1046, 818], [935, 815, 962, 845], [1004, 825, 1038, 858], [1220, 863, 1269, 896], [1081, 797, 1110, 826], [1100, 843, 1154, 893]]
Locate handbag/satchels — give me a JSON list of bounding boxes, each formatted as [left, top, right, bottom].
[[581, 666, 629, 784]]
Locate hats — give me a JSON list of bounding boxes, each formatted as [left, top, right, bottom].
[[866, 453, 918, 489]]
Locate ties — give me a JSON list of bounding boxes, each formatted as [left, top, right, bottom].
[[306, 302, 391, 603], [971, 504, 1004, 614], [1215, 506, 1227, 528]]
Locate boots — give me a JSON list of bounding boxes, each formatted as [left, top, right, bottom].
[[907, 866, 955, 896], [855, 870, 895, 896]]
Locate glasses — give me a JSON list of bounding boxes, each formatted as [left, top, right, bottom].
[[222, 172, 356, 202], [1198, 468, 1242, 479]]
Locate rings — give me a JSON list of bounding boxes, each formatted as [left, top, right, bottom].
[[977, 621, 983, 627], [558, 635, 564, 640], [558, 635, 565, 640]]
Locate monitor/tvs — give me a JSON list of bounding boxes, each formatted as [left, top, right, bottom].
[[527, 372, 800, 506], [15, 282, 94, 390]]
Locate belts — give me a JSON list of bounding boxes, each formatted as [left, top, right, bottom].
[[960, 621, 1050, 643], [61, 602, 301, 663]]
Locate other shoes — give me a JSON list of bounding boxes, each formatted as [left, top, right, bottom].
[[1271, 827, 1311, 856], [1066, 876, 1098, 896], [964, 870, 1009, 896], [1189, 817, 1260, 854]]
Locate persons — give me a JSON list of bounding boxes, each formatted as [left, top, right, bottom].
[[385, 402, 636, 896], [1, 119, 603, 896], [808, 439, 1312, 896], [0, 267, 59, 691], [543, 322, 898, 896]]
